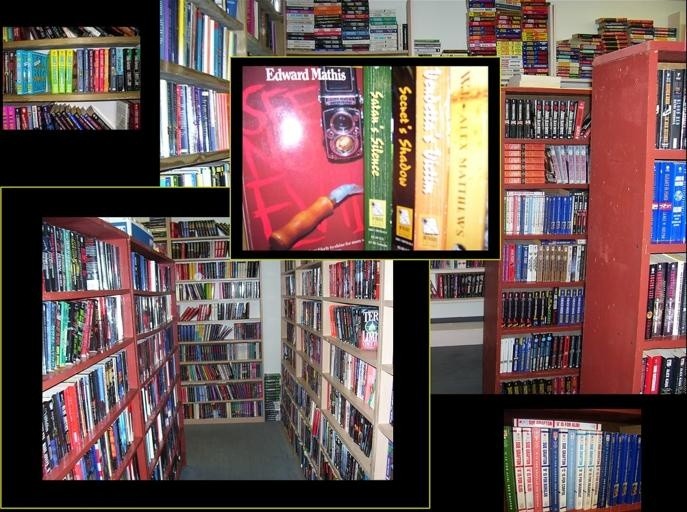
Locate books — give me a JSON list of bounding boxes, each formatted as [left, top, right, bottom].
[[160, 0, 412, 190], [363, 63, 488, 251], [499, 416, 642, 511], [412, 1, 685, 396], [43, 214, 394, 479], [0, 25, 141, 132]]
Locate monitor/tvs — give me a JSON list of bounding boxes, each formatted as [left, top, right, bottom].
[[231, 57, 503, 261]]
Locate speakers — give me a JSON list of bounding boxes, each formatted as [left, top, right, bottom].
[[318, 67, 364, 164]]
